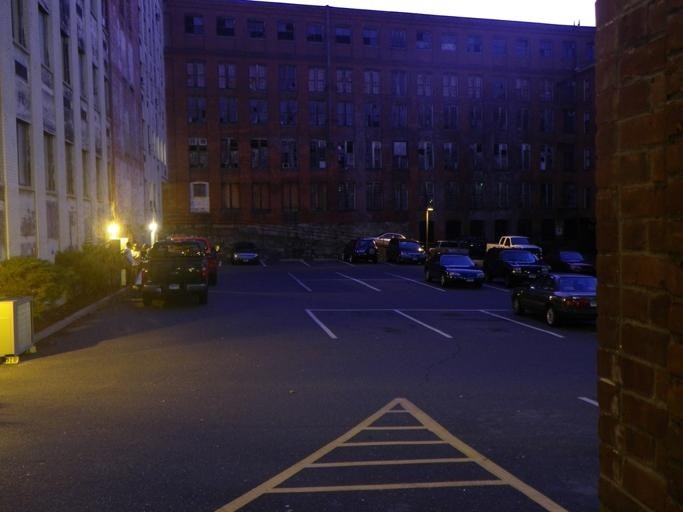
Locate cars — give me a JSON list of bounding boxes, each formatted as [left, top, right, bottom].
[[229, 240, 260, 265], [344, 230, 598, 330]]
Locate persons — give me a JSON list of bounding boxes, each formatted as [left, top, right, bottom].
[[124, 242, 148, 288]]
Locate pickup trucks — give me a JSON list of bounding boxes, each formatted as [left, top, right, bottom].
[[140, 238, 212, 307]]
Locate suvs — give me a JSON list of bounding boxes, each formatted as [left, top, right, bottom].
[[175, 234, 218, 286]]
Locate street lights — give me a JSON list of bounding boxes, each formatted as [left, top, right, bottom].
[[425, 207, 436, 252]]
